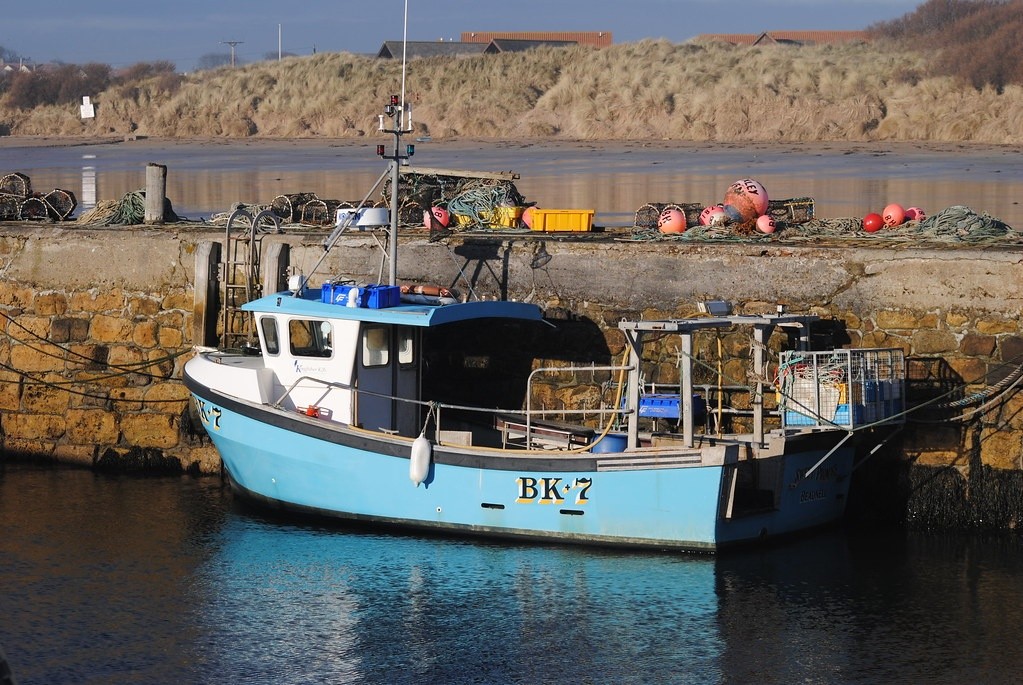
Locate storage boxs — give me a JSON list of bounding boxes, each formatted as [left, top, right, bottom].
[[621, 394, 701, 418], [321, 283, 399, 310], [456, 207, 523, 228], [786, 378, 902, 425], [529, 209, 594, 231]]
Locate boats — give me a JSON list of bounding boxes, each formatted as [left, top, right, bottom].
[[185, 0, 904, 551], [417, 137, 432, 142]]
